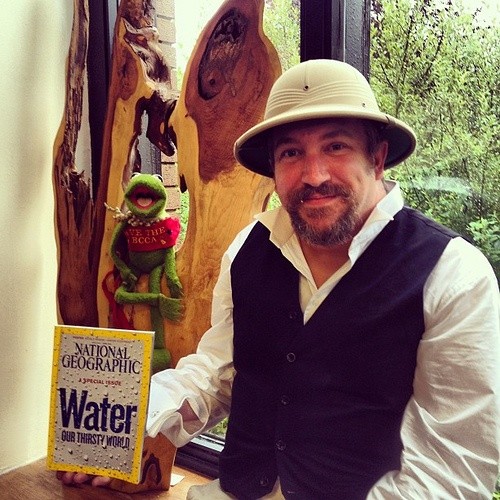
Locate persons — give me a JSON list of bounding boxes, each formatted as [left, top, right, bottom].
[[51, 57, 500, 500]]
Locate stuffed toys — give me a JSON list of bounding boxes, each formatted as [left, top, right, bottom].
[[108, 171, 188, 368]]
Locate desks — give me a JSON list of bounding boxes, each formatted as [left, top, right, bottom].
[[1, 446, 231, 500]]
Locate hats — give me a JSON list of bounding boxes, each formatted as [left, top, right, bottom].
[[234, 58, 418, 180]]
